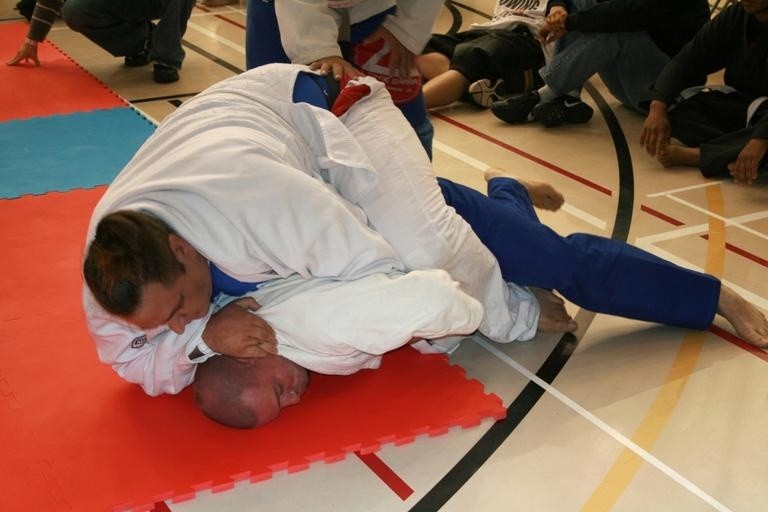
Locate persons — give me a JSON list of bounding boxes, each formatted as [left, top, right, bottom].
[[246, 1, 445, 163], [6, 1, 196, 84], [80, 61, 578, 399], [197, 171, 767, 428], [417, 0, 768, 186]]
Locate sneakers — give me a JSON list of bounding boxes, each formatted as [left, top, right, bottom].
[[126, 22, 157, 66], [154, 64, 179, 84], [466, 78, 593, 127]]
